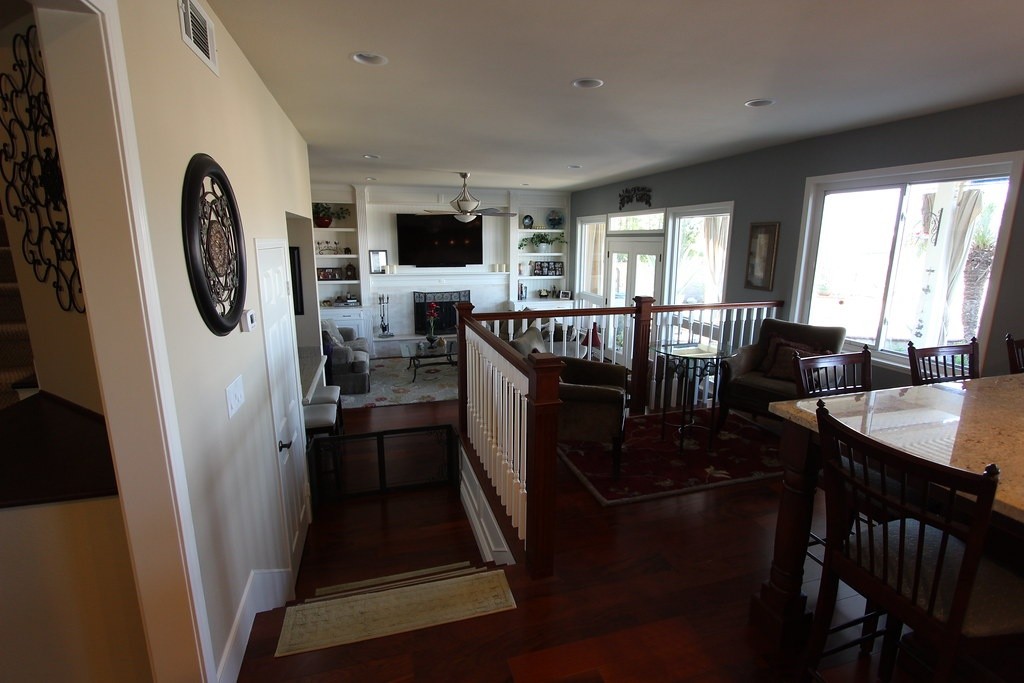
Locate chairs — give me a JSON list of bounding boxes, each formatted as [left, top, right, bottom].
[[792, 332, 1024, 683], [710, 317, 847, 440], [510, 326, 628, 484]]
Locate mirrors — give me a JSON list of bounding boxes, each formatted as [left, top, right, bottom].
[[368, 250, 389, 274]]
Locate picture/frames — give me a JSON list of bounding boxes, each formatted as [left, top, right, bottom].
[[317, 267, 342, 280], [533, 260, 564, 276], [560, 290, 571, 299], [744, 221, 781, 292]]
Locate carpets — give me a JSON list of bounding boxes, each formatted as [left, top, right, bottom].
[[340, 355, 458, 409], [557, 406, 785, 507], [275, 561, 518, 659]]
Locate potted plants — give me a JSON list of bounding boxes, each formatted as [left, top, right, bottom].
[[518, 232, 568, 253], [312, 202, 351, 228]]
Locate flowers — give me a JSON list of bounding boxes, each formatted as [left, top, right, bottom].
[[426, 302, 439, 327]]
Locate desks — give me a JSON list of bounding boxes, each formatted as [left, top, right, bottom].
[[646, 339, 739, 455], [761, 372, 1024, 655]]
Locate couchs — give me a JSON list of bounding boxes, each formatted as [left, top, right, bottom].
[[319, 319, 371, 395], [491, 320, 588, 358]]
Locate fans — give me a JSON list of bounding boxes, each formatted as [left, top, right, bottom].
[[416, 172, 517, 217]]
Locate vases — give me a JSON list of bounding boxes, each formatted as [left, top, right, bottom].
[[426, 327, 438, 349]]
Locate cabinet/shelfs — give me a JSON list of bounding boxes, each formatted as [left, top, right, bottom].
[[311, 202, 363, 339], [509, 190, 574, 325]]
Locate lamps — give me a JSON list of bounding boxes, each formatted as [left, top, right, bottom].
[[455, 215, 477, 223]]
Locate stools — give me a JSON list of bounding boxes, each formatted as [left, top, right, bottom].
[[303, 385, 345, 472]]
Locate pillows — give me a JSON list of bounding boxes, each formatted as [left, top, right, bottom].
[[499, 306, 572, 342], [760, 333, 834, 380]]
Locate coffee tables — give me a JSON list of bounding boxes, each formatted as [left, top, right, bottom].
[[399, 340, 458, 383]]
